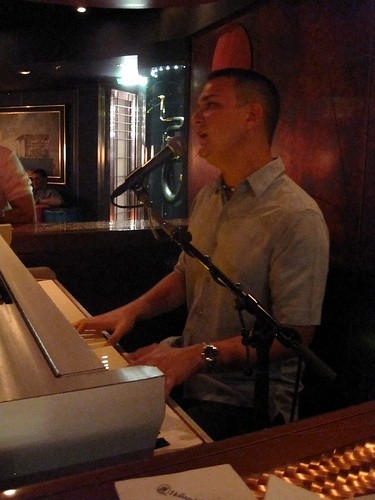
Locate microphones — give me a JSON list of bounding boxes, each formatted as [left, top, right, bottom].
[[109, 137, 185, 201]]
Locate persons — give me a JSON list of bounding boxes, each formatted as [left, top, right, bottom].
[[0, 146, 39, 226], [29, 169, 65, 208], [69, 69, 333, 441]]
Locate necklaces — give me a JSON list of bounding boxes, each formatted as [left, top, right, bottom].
[[221, 183, 237, 194]]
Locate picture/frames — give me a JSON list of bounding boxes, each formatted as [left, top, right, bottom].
[[1, 105, 66, 185]]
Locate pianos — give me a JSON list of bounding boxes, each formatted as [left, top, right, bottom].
[[0, 223, 208, 486]]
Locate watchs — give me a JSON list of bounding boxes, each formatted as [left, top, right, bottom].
[[202, 342, 221, 373]]
